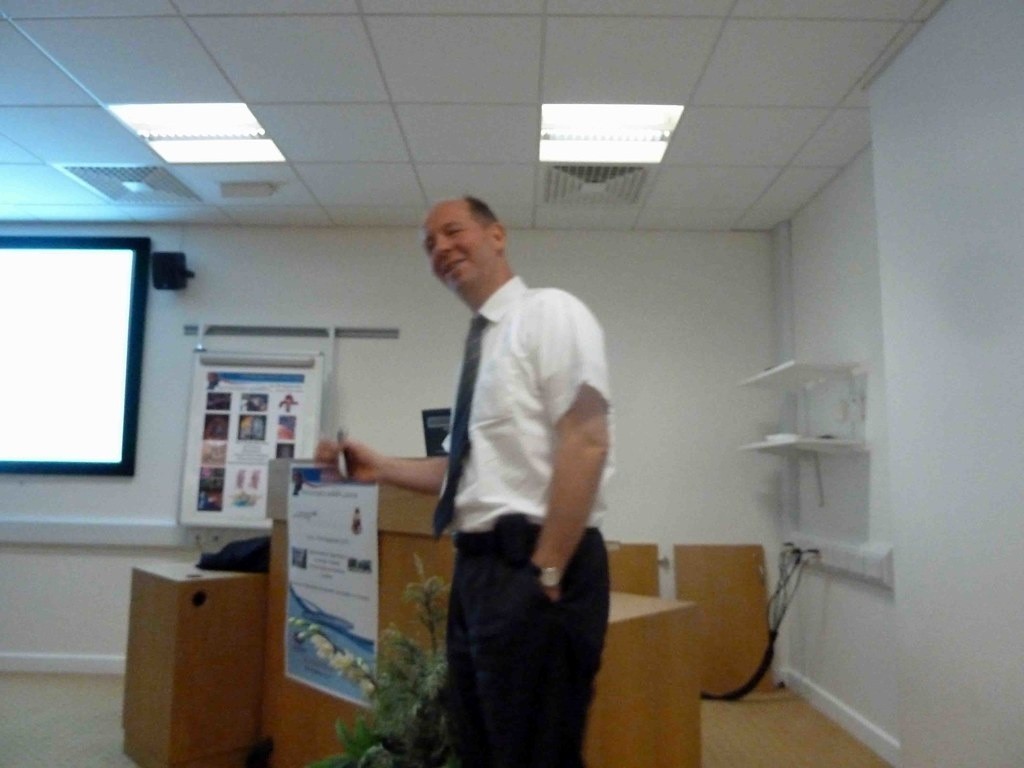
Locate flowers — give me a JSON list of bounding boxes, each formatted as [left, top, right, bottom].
[[287, 551, 449, 768]]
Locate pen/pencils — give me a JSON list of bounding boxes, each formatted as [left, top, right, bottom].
[[334, 428, 353, 482]]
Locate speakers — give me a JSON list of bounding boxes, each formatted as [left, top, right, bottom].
[[151, 251, 190, 293]]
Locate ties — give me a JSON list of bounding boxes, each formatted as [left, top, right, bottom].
[[432, 315, 488, 542]]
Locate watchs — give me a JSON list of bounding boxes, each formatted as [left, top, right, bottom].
[[529, 560, 561, 587]]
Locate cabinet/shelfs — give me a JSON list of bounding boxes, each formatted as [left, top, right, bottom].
[[739, 358, 865, 456]]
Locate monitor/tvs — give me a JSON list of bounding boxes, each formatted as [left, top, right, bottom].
[[422, 408, 452, 457]]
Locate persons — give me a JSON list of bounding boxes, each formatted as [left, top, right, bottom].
[[317, 197, 612, 768]]
